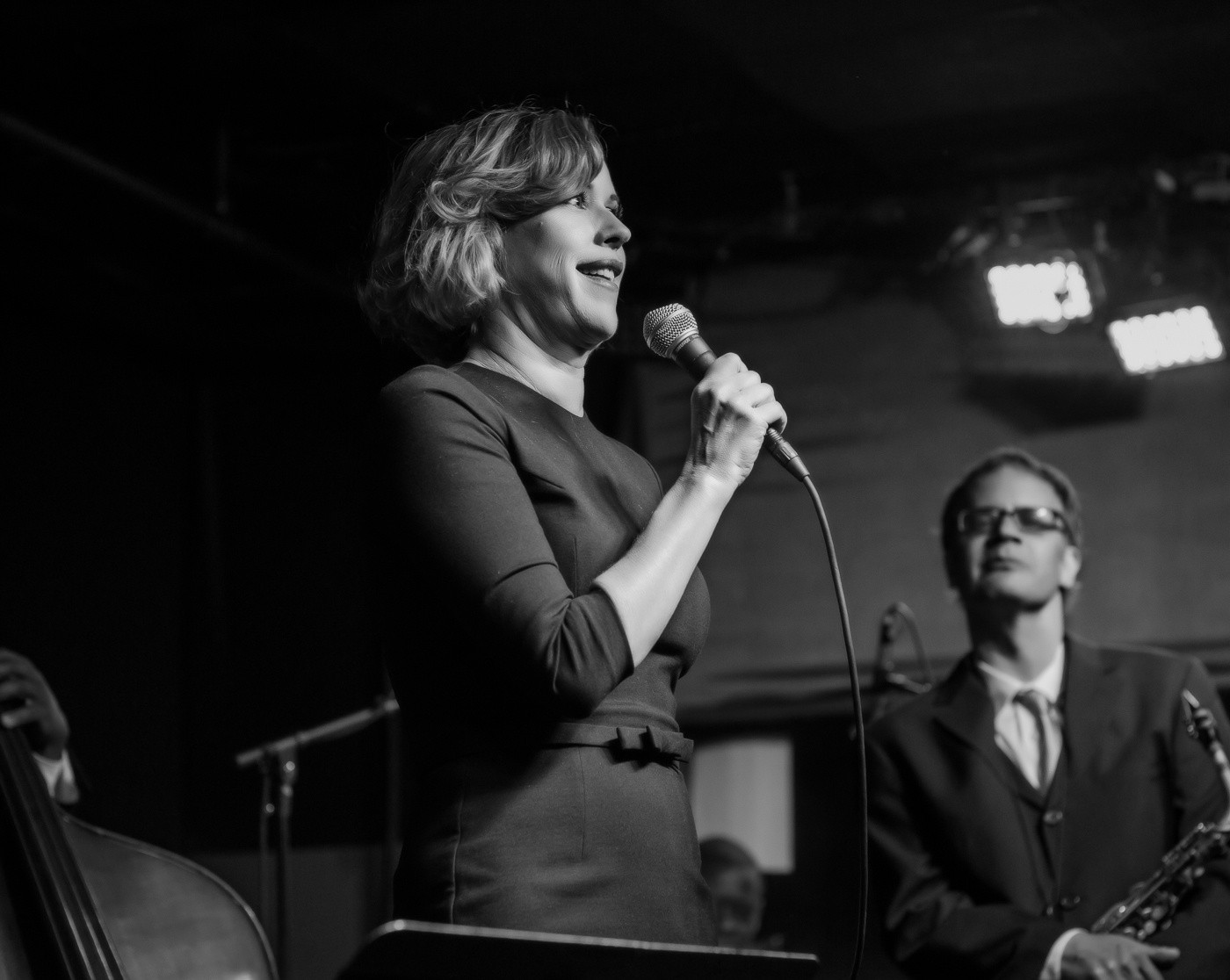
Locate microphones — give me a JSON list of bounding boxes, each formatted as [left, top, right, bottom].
[[644, 302, 810, 481]]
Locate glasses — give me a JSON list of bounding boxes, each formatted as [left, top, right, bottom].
[[951, 506, 1077, 546]]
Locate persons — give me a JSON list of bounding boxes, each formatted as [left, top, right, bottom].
[[364, 107, 788, 955], [3, 645, 84, 811], [837, 446, 1230, 979]]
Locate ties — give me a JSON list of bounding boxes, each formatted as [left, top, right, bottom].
[[1012, 689, 1053, 799]]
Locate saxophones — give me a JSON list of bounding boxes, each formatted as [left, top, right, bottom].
[[1087, 686, 1230, 939]]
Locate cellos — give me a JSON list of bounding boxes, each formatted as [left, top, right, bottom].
[[0, 698, 282, 980]]
